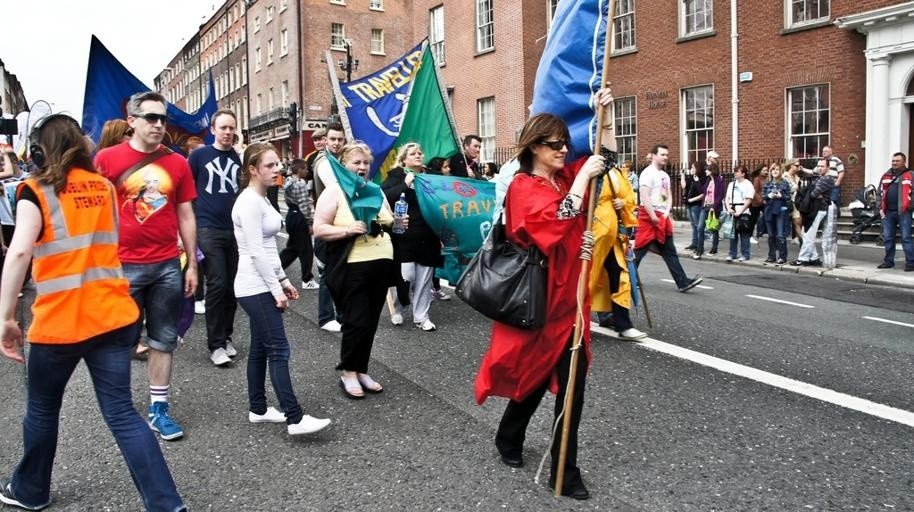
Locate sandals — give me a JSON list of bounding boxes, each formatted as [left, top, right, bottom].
[[357, 372, 384, 393], [339, 374, 364, 398]]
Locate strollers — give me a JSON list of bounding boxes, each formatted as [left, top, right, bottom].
[[849, 184, 885, 247]]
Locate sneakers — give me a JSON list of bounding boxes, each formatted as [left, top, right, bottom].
[[679, 275, 703, 292], [685, 245, 821, 266], [598, 315, 614, 332], [431, 288, 451, 301], [618, 328, 648, 340], [416, 319, 437, 331], [223, 339, 237, 357], [249, 406, 287, 423], [320, 319, 341, 333], [0, 477, 53, 510], [301, 278, 320, 290], [193, 300, 206, 315], [147, 401, 184, 440], [210, 347, 232, 366], [749, 236, 759, 244], [287, 414, 331, 436]]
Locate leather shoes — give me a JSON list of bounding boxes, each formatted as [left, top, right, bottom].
[[877, 261, 891, 268], [391, 311, 403, 327], [502, 455, 523, 468], [571, 479, 589, 499], [904, 265, 914, 271]]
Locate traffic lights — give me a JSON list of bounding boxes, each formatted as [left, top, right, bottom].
[[288, 103, 296, 138]]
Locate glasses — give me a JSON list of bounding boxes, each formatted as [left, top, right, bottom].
[[123, 129, 133, 137], [135, 112, 167, 124], [540, 139, 570, 150]]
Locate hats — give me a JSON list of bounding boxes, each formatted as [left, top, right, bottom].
[[706, 150, 719, 159], [786, 158, 799, 166], [310, 128, 326, 138]]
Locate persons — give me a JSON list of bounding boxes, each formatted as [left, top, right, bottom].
[[3, 93, 706, 509], [680, 146, 844, 269], [877, 152, 914, 271]]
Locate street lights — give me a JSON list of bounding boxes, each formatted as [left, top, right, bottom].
[[162, 65, 191, 116]]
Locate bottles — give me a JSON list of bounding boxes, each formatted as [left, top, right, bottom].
[[391, 193, 408, 235], [624, 233, 636, 263], [772, 184, 778, 195]]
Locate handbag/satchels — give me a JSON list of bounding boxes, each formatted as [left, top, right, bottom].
[[705, 210, 721, 231], [722, 211, 736, 240], [454, 171, 547, 331], [731, 213, 755, 235]]
